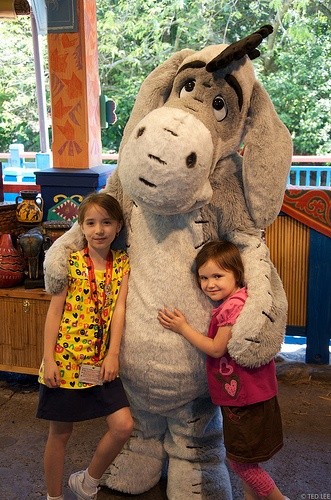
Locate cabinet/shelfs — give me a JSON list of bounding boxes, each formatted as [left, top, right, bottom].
[[0, 288, 53, 375]]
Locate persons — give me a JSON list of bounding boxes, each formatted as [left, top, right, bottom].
[[36, 193, 134, 500], [158, 241, 289, 500]]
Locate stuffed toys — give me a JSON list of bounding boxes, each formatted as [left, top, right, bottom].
[[43, 25, 294, 500]]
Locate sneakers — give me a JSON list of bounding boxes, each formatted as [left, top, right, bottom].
[[69, 470, 101, 500]]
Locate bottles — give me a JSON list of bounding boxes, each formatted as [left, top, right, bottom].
[[0, 230, 24, 285]]
